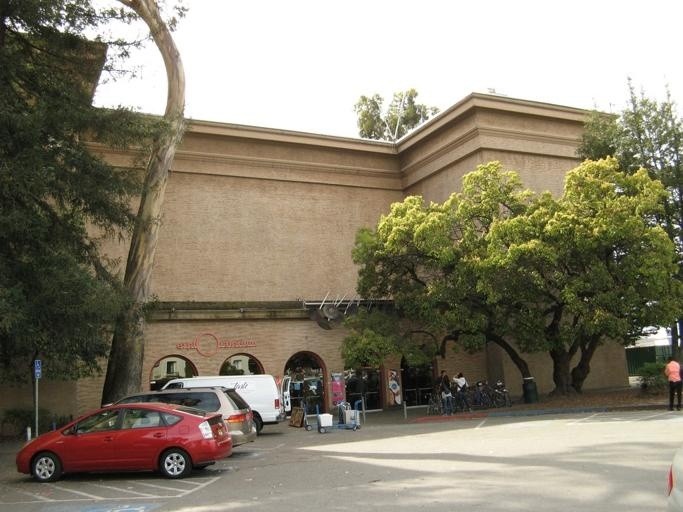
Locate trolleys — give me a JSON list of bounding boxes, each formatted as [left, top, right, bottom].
[[313, 403, 334, 434], [301, 402, 318, 431], [334, 399, 361, 431]]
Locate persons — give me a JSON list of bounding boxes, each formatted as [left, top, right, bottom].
[[663, 356, 682, 411], [435, 369, 455, 416], [452, 371, 473, 414]]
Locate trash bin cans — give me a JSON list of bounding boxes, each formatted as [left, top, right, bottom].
[[523, 376, 537, 403]]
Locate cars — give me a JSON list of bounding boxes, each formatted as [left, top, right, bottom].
[[664, 448, 683, 512], [15, 400, 234, 483]]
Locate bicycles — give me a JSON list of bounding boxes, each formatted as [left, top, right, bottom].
[[423, 377, 513, 417]]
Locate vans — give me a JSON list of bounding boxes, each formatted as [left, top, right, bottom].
[[158, 372, 288, 434], [92, 385, 256, 449]]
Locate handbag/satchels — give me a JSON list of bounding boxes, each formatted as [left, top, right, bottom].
[[443, 382, 450, 395], [457, 383, 465, 392]]
